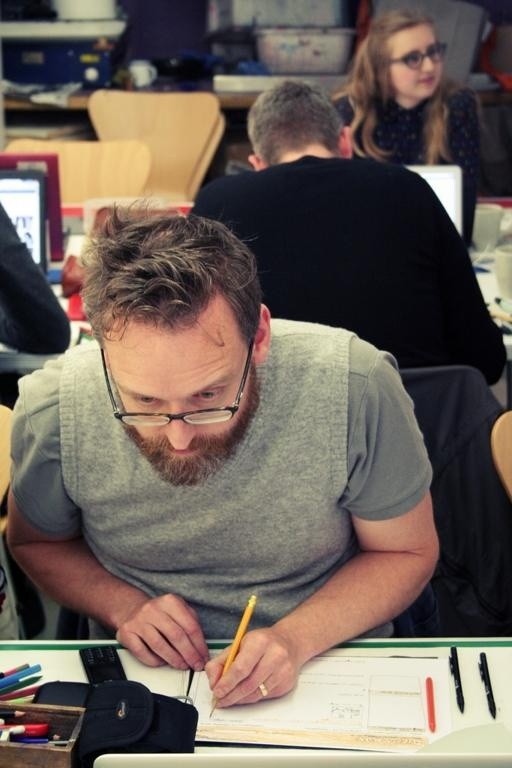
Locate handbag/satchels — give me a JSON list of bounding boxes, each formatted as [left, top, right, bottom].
[[33, 680, 198, 768]]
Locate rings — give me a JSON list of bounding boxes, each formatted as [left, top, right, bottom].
[[260, 685, 268, 698]]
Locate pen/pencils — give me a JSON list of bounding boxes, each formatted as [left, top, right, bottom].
[[477, 652, 496, 720], [208, 595, 257, 717], [448, 645, 464, 714], [427, 678, 435, 732], [0, 664, 68, 745]]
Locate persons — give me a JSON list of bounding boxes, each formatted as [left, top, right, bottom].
[[0, 202, 71, 409], [8, 201, 440, 708], [191, 77, 506, 387], [331, 5, 479, 247], [356, 0, 512, 78]]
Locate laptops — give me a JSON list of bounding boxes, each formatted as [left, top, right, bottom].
[[0, 169, 47, 278], [401, 160, 469, 255]]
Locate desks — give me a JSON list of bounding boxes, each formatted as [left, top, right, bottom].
[[1, 637, 512, 650], [0, 204, 512, 371]]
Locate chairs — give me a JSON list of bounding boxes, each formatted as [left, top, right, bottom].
[[398, 364, 502, 634], [4, 137, 153, 198], [88, 87, 227, 206], [490, 411, 512, 500]]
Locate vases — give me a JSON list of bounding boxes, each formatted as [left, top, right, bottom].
[[256, 26, 354, 75]]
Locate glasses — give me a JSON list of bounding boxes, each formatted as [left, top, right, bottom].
[[391, 40, 446, 69], [100, 339, 253, 427]]
